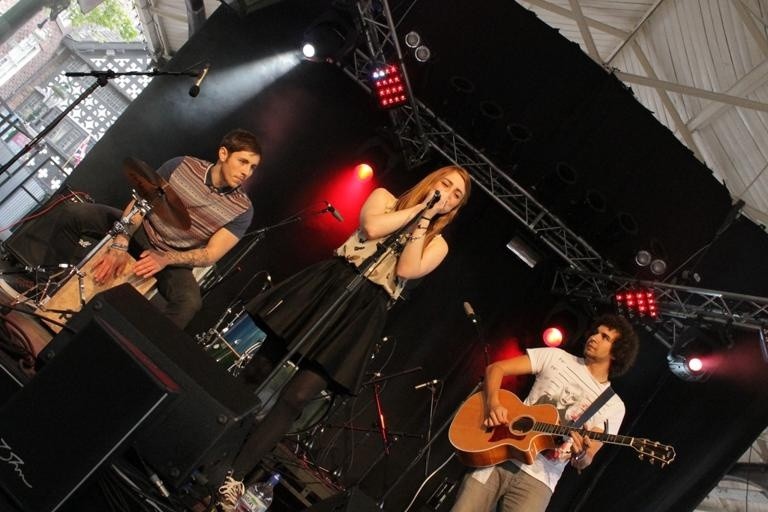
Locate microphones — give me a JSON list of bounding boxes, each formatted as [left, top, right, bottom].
[[433, 187, 442, 209], [462, 301, 478, 326], [328, 204, 344, 222], [188, 65, 211, 97], [412, 377, 440, 392]]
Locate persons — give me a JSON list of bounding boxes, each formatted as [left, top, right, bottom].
[[438, 311, 642, 512], [204, 163, 473, 510], [0, 126, 266, 330]]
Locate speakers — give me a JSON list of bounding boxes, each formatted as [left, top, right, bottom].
[[0, 311, 169, 511], [34, 284, 262, 511]]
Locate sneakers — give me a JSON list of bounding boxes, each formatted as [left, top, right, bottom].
[[220, 468, 246, 511]]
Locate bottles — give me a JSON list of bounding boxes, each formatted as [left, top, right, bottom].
[[231, 471, 282, 512]]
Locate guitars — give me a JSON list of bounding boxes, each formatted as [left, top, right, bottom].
[[448, 388, 676, 468]]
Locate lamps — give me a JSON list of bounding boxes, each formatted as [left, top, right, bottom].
[[296, 0, 768, 382]]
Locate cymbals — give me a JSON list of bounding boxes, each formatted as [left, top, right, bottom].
[[121, 157, 192, 230]]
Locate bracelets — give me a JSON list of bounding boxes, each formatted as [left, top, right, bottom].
[[406, 233, 425, 241], [419, 214, 431, 222], [415, 222, 428, 231], [109, 243, 130, 252]]
